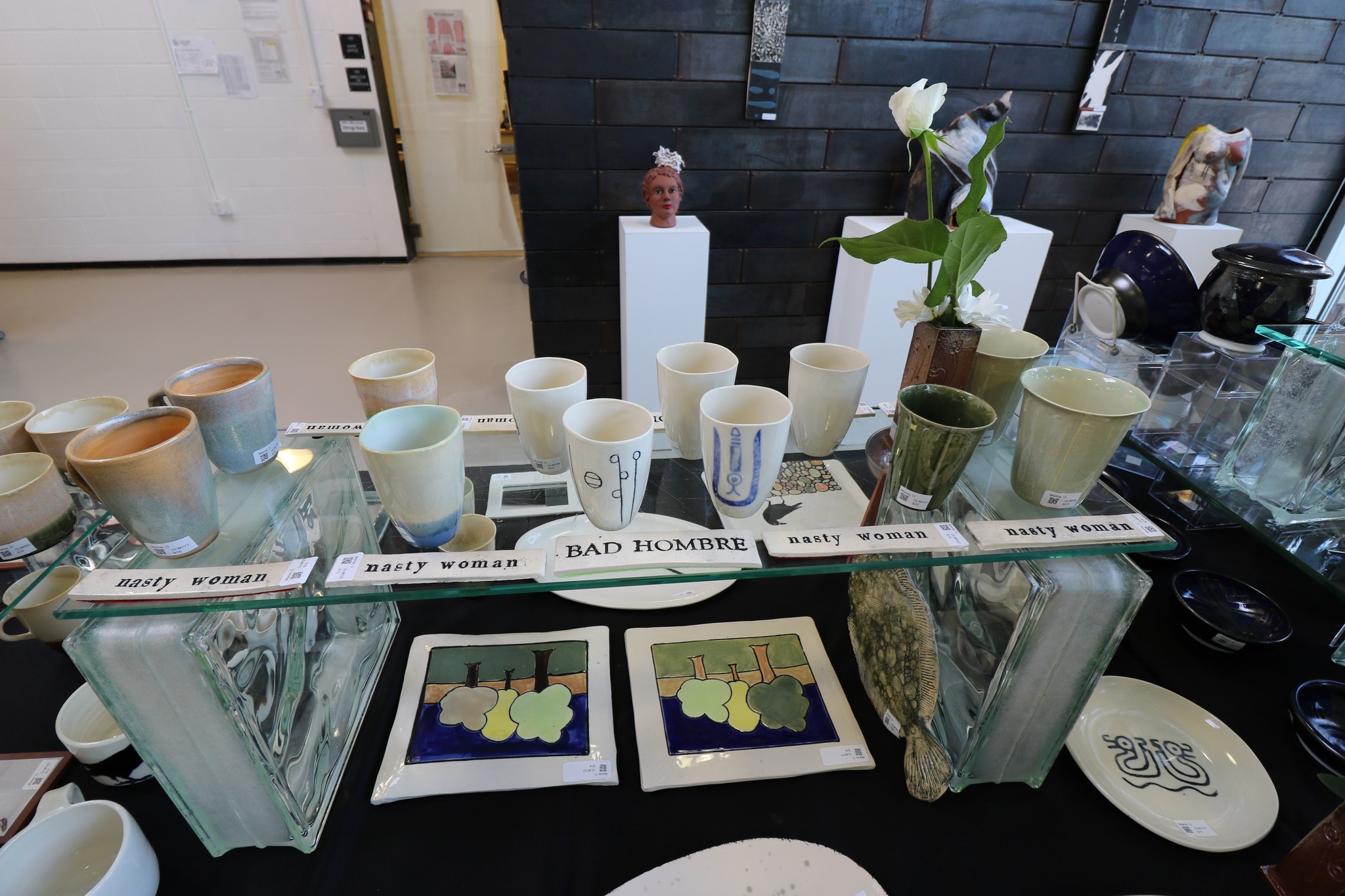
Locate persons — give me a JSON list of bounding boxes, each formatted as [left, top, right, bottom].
[[1153, 123, 1253, 225], [642, 166, 683, 227]]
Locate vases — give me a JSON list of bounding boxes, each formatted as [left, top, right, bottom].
[[888, 318, 982, 453]]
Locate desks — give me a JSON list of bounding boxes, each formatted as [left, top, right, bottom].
[[0, 420, 1180, 863]]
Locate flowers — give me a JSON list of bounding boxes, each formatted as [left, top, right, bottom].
[[817, 77, 1009, 327]]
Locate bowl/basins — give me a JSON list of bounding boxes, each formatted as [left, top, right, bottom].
[[866, 424, 898, 478], [55, 681, 155, 788], [1170, 569, 1292, 649], [1082, 471, 1130, 515], [1199, 244, 1334, 353], [0, 800, 159, 896], [1078, 231, 1198, 340], [1100, 503, 1192, 569], [1289, 680, 1345, 786]]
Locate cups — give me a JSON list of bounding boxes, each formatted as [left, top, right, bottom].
[[0, 566, 94, 643], [348, 348, 497, 553], [504, 357, 588, 475], [701, 386, 793, 515], [967, 326, 1050, 446], [657, 342, 739, 458], [149, 358, 280, 476], [563, 398, 654, 529], [24, 396, 129, 487], [0, 401, 79, 563], [65, 406, 220, 559], [892, 385, 997, 513], [788, 342, 869, 458], [1011, 366, 1152, 508]]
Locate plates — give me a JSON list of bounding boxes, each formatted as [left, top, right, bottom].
[[1064, 675, 1279, 852], [514, 513, 741, 609]]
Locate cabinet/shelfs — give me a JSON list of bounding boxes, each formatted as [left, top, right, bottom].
[[1050, 325, 1345, 607]]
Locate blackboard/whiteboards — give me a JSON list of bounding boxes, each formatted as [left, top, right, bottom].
[[0, 1, 418, 266]]
